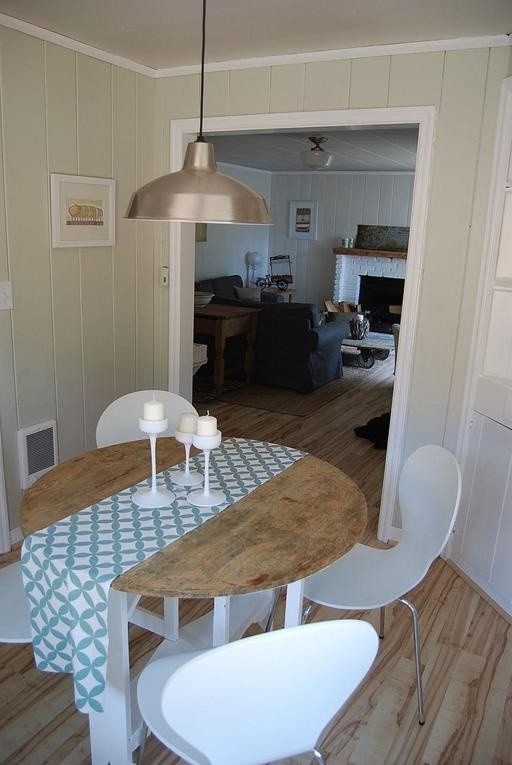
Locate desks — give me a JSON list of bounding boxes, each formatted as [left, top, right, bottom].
[[20, 435, 369, 763], [194, 303, 256, 398]]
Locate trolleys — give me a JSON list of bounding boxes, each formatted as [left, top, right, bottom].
[[341, 340, 390, 369]]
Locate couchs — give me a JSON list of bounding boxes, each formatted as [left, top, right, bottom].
[[208, 295, 343, 394], [195, 275, 243, 344]]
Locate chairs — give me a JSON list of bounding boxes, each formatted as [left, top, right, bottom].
[[136, 620, 378, 764], [95, 389, 202, 451], [263, 442, 462, 727]]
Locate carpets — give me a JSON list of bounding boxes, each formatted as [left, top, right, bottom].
[[193, 345, 395, 418]]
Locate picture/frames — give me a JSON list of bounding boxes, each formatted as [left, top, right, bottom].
[[50, 173, 116, 249], [288, 200, 318, 240]]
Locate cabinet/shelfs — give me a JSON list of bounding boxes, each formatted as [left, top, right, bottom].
[[447, 411, 512, 616]]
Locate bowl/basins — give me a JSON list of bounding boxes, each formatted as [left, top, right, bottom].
[[194, 291, 215, 308]]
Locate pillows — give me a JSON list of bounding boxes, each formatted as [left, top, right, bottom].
[[234, 287, 260, 302]]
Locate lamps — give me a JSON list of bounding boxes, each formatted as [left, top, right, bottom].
[[248, 252, 262, 283], [302, 136, 333, 170], [122, 0, 276, 226]]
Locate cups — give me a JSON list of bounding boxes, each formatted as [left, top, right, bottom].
[[342, 237, 354, 249]]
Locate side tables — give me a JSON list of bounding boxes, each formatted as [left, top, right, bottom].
[[261, 288, 296, 303]]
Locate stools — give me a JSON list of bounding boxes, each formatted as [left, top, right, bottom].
[[350, 319, 369, 340]]
